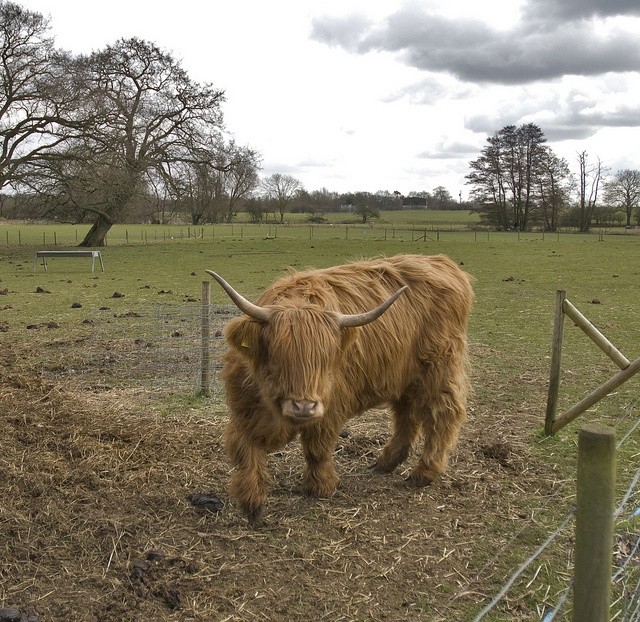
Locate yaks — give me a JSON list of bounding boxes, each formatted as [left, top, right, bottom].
[[204, 252, 475, 518]]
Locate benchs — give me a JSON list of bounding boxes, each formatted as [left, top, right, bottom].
[[32, 249, 106, 274]]
